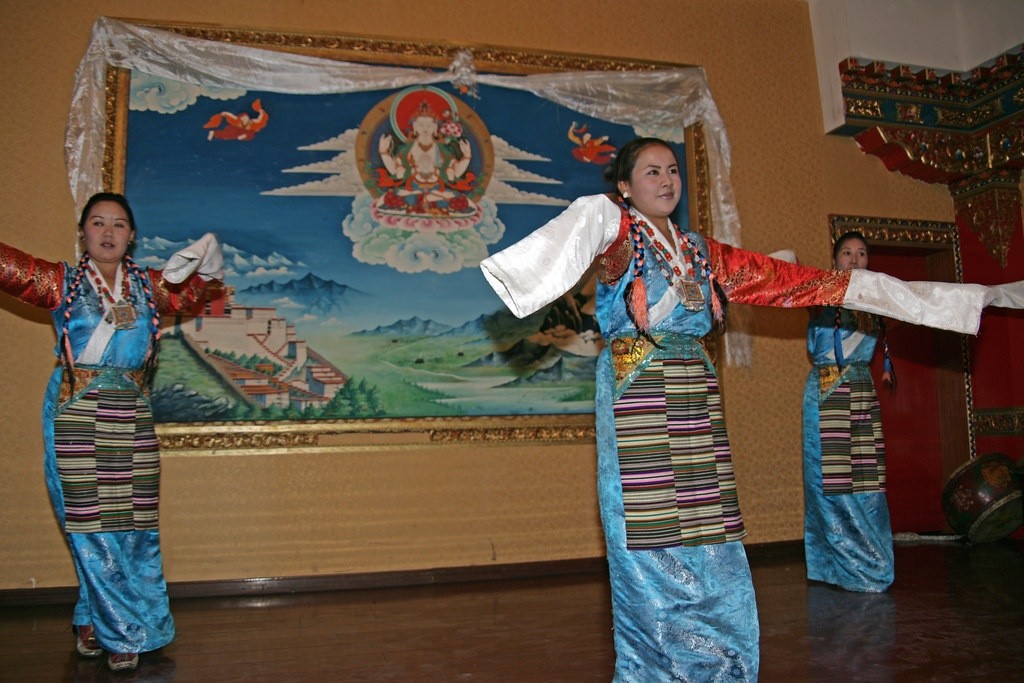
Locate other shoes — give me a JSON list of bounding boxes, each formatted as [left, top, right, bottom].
[[76, 624, 102, 657], [108, 652, 140, 671]]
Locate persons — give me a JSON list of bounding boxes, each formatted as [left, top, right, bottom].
[[0, 191, 227, 671], [480, 138, 996, 683], [766, 231, 1024, 594]]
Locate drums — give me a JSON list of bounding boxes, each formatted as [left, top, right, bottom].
[[939, 450, 1024, 546]]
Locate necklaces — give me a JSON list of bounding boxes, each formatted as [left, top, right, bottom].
[[638, 221, 706, 290], [85, 263, 129, 324]]
